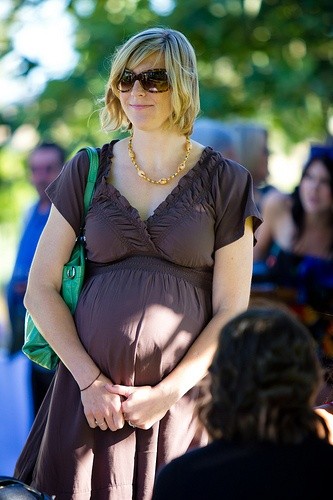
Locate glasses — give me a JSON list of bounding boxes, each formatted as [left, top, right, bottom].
[[116, 67, 171, 94]]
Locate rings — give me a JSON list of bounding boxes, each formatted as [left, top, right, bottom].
[[96, 421, 107, 428], [128, 420, 135, 428]]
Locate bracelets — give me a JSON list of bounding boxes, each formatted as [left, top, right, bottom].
[[80, 372, 102, 391]]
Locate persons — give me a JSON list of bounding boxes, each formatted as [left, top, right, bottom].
[[5, 143, 66, 418], [151, 307, 333, 500], [13, 27, 264, 500], [191, 118, 333, 411]]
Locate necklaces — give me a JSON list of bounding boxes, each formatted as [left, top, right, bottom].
[[126, 129, 192, 183]]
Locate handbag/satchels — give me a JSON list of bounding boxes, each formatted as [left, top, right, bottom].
[[21, 146, 99, 372]]
